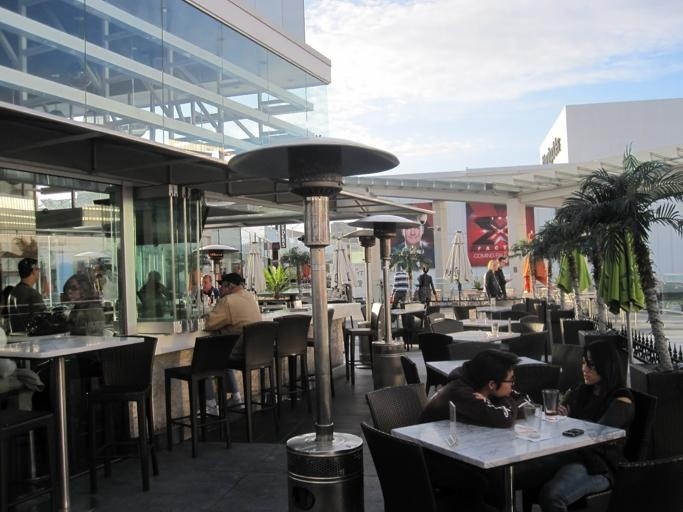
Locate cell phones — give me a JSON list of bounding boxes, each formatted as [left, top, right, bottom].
[[562, 428, 585, 438]]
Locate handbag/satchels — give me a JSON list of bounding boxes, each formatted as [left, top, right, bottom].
[[496, 292, 505, 301]]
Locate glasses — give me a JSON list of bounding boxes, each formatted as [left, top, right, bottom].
[[489, 375, 516, 387]]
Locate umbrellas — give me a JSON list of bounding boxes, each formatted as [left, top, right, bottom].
[[443, 229, 473, 299], [558, 251, 593, 318], [244, 242, 266, 292], [331, 235, 360, 302], [524, 230, 548, 293], [595, 222, 646, 362]]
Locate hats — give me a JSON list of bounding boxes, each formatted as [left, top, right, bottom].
[[215, 272, 244, 286]]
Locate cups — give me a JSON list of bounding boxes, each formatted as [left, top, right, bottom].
[[524, 404, 543, 437], [490, 320, 501, 338], [542, 389, 560, 420]]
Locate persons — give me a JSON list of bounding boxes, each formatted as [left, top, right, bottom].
[[8, 257, 48, 332], [140, 270, 171, 313], [484, 259, 504, 300], [390, 214, 435, 268], [416, 350, 531, 512], [197, 272, 264, 419], [0, 326, 45, 411], [494, 339, 636, 512], [412, 264, 438, 304], [494, 257, 513, 298], [62, 272, 106, 336], [200, 275, 220, 303], [391, 263, 410, 309]]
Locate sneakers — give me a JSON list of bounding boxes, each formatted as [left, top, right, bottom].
[[196, 405, 219, 420], [226, 396, 246, 414]]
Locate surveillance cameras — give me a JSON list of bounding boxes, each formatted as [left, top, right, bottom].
[[419, 214, 427, 224]]
